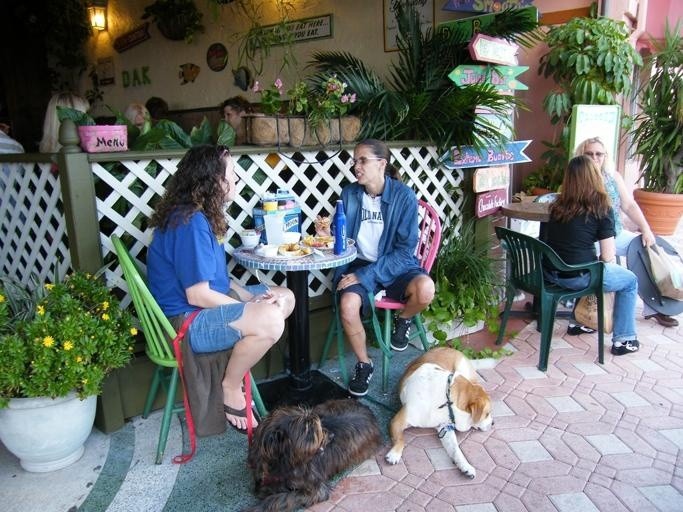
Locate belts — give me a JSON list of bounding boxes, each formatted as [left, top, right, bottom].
[[540, 263, 589, 278]]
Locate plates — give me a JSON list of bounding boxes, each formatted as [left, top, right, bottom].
[[255, 244, 315, 261], [299, 235, 355, 251]]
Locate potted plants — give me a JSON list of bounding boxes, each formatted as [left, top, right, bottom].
[[527, 18, 634, 195], [622, 23, 682, 236], [409, 213, 518, 352]]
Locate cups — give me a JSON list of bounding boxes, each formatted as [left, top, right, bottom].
[[262, 213, 285, 244]]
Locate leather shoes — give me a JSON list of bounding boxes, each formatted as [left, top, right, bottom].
[[567, 323, 595, 335], [645, 313, 678, 326], [611, 340, 639, 356]]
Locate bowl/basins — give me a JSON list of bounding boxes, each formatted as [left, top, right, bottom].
[[282, 231, 301, 245], [333, 200, 348, 255], [239, 233, 261, 249]]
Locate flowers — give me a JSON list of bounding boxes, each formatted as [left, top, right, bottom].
[[256, 76, 357, 117], [0, 271, 137, 400]]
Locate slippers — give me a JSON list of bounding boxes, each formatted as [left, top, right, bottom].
[[224, 404, 262, 433]]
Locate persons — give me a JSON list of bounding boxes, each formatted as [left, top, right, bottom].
[[579, 138, 678, 327], [311, 136, 436, 398], [1, 91, 254, 153], [540, 157, 641, 356], [145, 145, 296, 436]]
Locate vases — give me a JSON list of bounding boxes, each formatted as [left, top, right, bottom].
[[238, 113, 363, 146], [1, 390, 98, 471]]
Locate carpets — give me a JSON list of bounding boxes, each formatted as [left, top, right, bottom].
[[0, 337, 427, 511]]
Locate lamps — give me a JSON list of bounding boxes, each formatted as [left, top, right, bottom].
[[86, 1, 110, 35]]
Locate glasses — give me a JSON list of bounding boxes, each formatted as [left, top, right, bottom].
[[350, 157, 379, 165]]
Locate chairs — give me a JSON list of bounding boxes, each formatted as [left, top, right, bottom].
[[533, 190, 622, 265], [110, 234, 274, 465], [493, 227, 606, 370], [319, 193, 441, 391]]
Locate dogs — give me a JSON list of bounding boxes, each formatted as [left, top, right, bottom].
[[384, 345, 496, 480], [242, 397, 385, 512]]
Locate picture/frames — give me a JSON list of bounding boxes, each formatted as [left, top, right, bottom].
[[383, 2, 438, 54]]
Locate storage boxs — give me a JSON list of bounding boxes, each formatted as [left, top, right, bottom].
[[75, 125, 127, 153], [250, 206, 303, 245]]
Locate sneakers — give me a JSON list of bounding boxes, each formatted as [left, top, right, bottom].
[[349, 358, 373, 396], [391, 312, 412, 352]]
